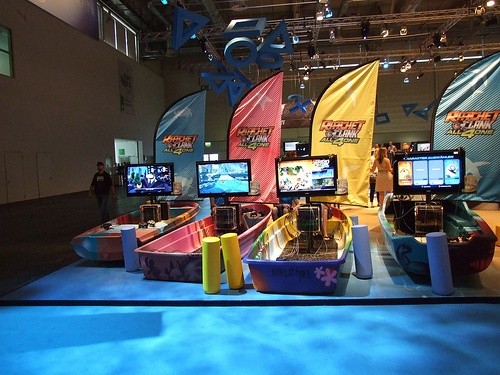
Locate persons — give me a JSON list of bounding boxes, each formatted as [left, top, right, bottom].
[[88, 162, 115, 224], [370, 149, 393, 205], [111, 161, 129, 187], [373, 141, 413, 167], [369, 151, 380, 207]]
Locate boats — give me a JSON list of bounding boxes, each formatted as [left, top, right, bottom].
[[246, 206, 353, 294], [71, 199, 200, 263], [134, 200, 270, 286], [377, 190, 495, 278]]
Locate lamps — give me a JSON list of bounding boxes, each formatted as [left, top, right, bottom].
[[315, 0, 332, 20], [400, 23, 408, 35], [362, 21, 371, 39], [329, 28, 335, 40], [381, 23, 391, 37], [290, 31, 320, 60], [399, 60, 424, 83], [474, 0, 497, 28], [428, 33, 447, 48], [200, 38, 213, 62], [257, 35, 282, 43], [289, 64, 311, 89], [384, 56, 390, 69], [459, 53, 464, 62]]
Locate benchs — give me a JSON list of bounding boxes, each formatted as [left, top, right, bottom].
[[445, 213, 469, 237]]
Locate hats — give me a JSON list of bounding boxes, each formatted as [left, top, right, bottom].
[[97, 162, 104, 166]]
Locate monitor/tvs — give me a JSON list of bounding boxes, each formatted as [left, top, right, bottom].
[[394, 148, 465, 203], [275, 155, 338, 203], [123, 163, 175, 203], [196, 159, 253, 206]]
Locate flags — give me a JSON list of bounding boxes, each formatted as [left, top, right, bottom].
[[228, 72, 283, 204], [154, 91, 206, 201], [431, 52, 500, 202], [309, 60, 380, 207]]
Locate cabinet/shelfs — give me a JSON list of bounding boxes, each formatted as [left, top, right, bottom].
[[215, 207, 237, 230], [414, 204, 443, 234]]
